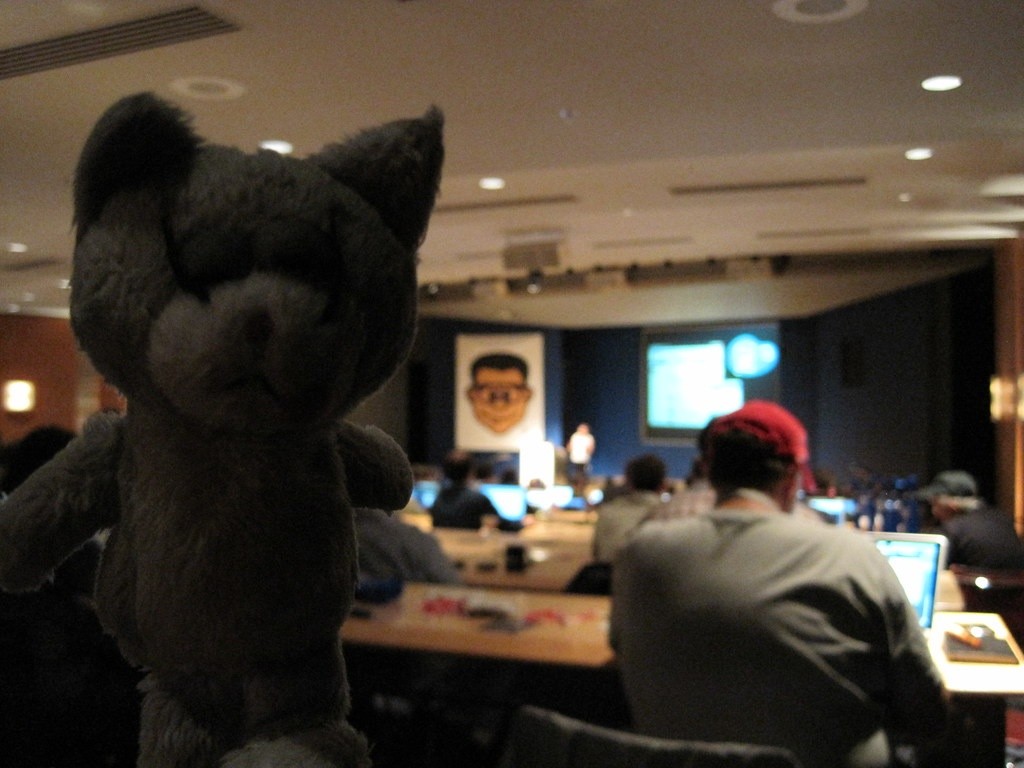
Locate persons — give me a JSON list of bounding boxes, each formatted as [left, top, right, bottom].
[[592, 455, 667, 561], [917, 470, 1024, 569], [0, 422, 146, 768], [647, 419, 822, 522], [430, 449, 534, 532], [352, 508, 459, 584], [610, 396, 951, 768], [566, 422, 595, 485]]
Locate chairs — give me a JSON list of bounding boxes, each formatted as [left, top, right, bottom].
[[506, 707, 798, 768]]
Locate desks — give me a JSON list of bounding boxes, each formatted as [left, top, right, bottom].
[[337, 476, 1024, 768]]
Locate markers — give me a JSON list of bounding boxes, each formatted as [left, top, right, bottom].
[[453, 560, 496, 572], [947, 627, 982, 648]]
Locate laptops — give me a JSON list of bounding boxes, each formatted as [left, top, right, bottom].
[[410, 480, 440, 509], [807, 494, 857, 527], [863, 531, 947, 641], [480, 482, 587, 524]]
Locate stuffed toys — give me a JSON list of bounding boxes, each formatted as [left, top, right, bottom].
[[0, 92, 446, 768]]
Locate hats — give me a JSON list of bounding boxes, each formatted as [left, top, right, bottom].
[[919, 471, 974, 498], [706, 402, 815, 490]]
